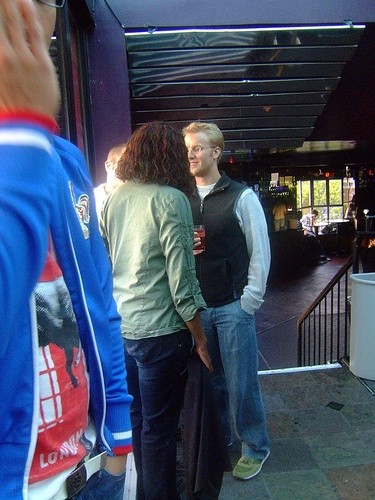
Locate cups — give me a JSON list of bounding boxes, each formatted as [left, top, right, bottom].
[[193, 225, 206, 255]]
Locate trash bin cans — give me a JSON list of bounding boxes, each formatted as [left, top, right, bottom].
[[349, 273, 375, 381]]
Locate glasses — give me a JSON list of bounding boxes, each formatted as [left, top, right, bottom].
[[187, 145, 216, 152], [34, 0, 65, 7]]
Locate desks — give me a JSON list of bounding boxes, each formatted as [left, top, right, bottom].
[[329, 219, 350, 258], [304, 222, 331, 264]]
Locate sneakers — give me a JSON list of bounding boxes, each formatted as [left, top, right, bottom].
[[232, 450, 270, 480]]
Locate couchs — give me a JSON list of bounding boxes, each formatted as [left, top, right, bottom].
[[297, 227, 319, 264]]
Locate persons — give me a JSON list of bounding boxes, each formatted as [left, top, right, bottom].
[[93, 145, 126, 218], [0, 0, 133, 500], [100, 120, 233, 500], [299, 209, 331, 262], [182, 121, 270, 481]]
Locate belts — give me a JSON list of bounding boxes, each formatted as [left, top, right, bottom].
[[51, 451, 106, 500]]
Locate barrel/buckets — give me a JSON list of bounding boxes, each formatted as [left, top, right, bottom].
[[349, 273, 375, 381]]
